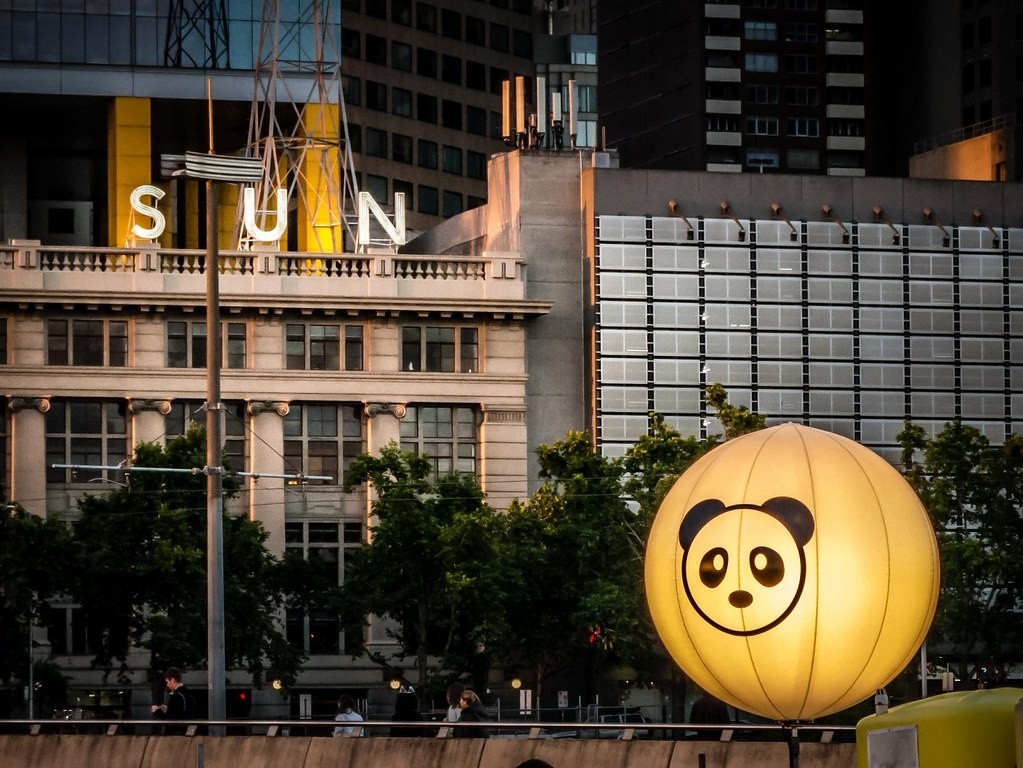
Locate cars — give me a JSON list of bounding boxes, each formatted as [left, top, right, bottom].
[[685, 718, 757, 740], [40, 704, 119, 732]]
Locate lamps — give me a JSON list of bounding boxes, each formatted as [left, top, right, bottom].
[[720, 200, 746, 243], [272, 680, 282, 690], [974, 209, 1000, 249], [511, 678, 521, 689], [642, 418, 942, 732], [769, 201, 798, 242], [873, 205, 900, 246], [822, 204, 851, 245], [390, 681, 401, 690], [668, 200, 694, 241], [924, 208, 950, 248]]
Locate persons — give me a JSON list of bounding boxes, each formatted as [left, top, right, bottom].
[[689, 693, 733, 741], [151, 667, 192, 735], [445, 684, 491, 739], [334, 696, 365, 738], [389, 693, 432, 738]]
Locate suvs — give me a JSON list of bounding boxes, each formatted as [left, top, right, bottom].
[[550, 707, 653, 740]]
